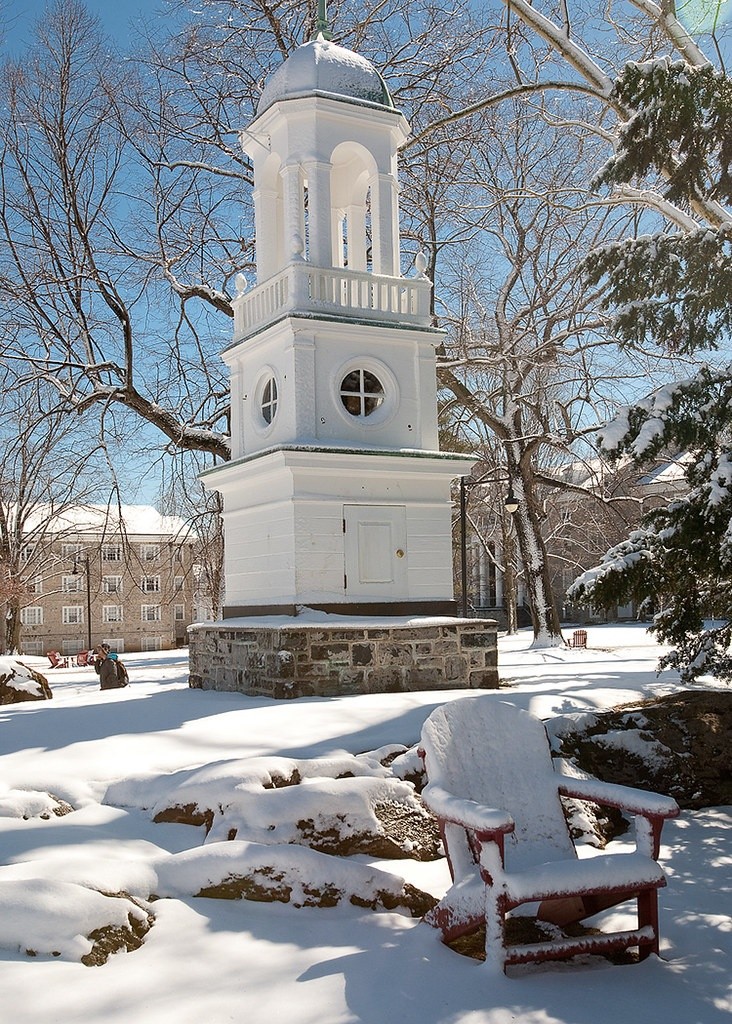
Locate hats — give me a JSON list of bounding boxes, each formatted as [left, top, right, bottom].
[[98, 643, 111, 655]]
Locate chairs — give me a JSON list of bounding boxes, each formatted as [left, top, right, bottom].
[[417, 697, 679, 983], [566, 630, 588, 649]]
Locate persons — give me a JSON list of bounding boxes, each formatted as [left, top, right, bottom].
[[95, 644, 125, 691]]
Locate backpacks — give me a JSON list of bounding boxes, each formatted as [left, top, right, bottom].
[[117, 659, 129, 688]]
